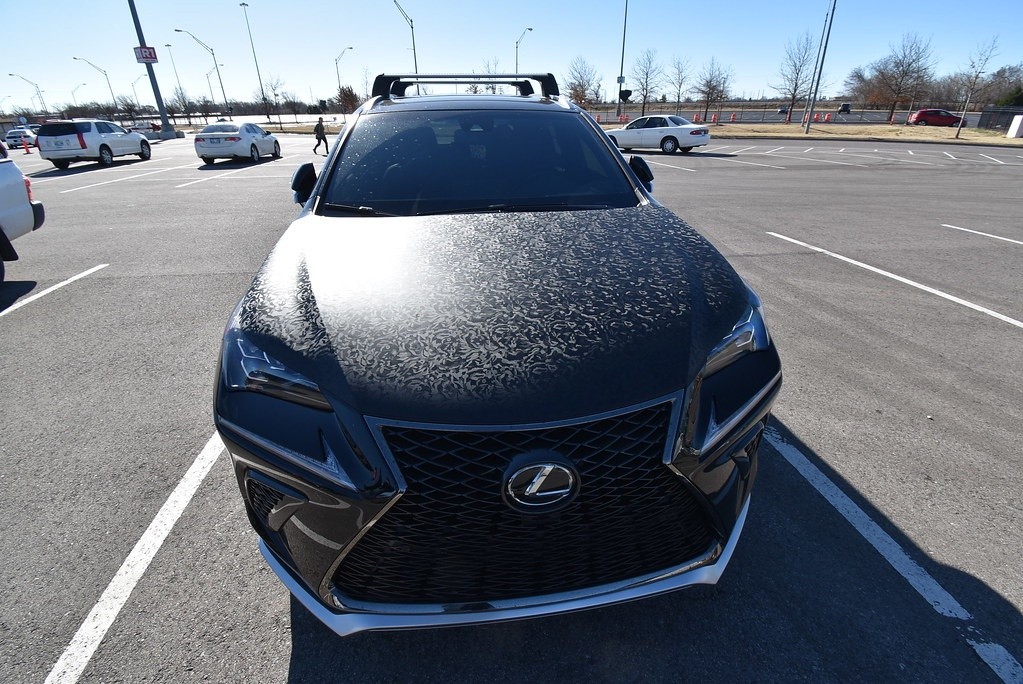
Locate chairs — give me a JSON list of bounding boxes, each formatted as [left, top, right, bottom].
[[387, 127, 443, 197]]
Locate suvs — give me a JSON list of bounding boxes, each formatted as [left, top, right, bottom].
[[209, 71, 786, 636], [36, 118, 152, 170], [838, 103, 851, 115]]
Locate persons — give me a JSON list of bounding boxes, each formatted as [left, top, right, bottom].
[[151, 123, 161, 131], [0, 139, 9, 159], [313, 117, 329, 155]]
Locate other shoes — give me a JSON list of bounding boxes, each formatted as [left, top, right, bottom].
[[326, 152, 329, 154], [313, 149, 317, 154]]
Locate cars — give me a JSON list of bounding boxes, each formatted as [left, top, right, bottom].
[[604, 115, 710, 155], [0, 140, 46, 284], [194, 122, 282, 166], [910, 108, 969, 129], [778, 106, 789, 114], [5, 124, 41, 149], [126, 122, 162, 132]]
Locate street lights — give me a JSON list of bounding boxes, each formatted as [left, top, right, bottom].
[[239, 2, 271, 122], [516, 28, 533, 96], [165, 44, 191, 125], [131, 74, 149, 122], [335, 46, 353, 123], [74, 57, 123, 128], [206, 63, 224, 120], [0, 74, 86, 118], [174, 29, 234, 122]]
